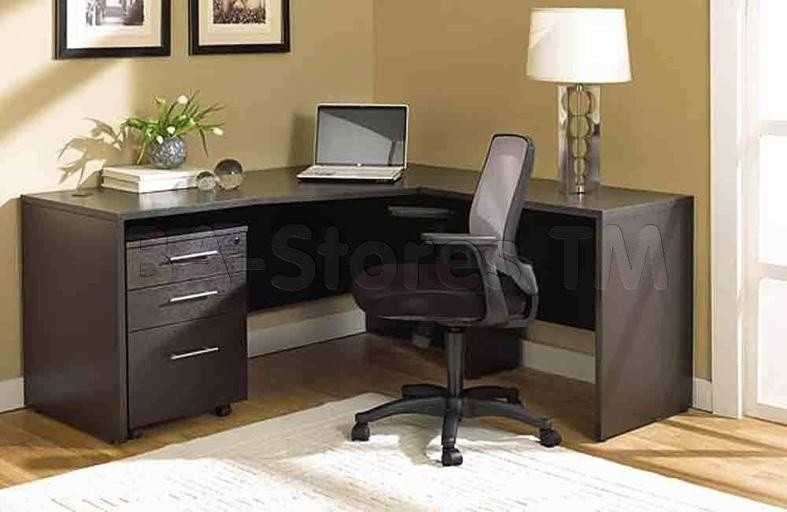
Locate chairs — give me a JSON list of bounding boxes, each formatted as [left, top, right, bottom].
[[352, 135, 561, 465]]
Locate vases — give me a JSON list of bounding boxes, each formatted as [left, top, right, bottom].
[[147, 137, 187, 169]]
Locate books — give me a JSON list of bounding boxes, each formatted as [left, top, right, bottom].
[[100, 167, 201, 194]]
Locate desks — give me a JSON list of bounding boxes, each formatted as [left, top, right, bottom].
[[20, 163, 694, 443]]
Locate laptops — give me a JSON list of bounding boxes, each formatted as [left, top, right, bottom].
[[296, 103, 409, 184]]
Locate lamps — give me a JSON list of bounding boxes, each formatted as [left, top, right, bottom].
[[527, 8, 633, 195]]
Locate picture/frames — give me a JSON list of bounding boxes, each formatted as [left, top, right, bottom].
[[55, 0, 170, 60], [188, 0, 289, 55]]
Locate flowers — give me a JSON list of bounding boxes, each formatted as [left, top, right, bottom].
[[120, 90, 227, 165]]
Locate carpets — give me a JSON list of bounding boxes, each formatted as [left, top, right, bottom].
[[1, 392, 786, 512]]
